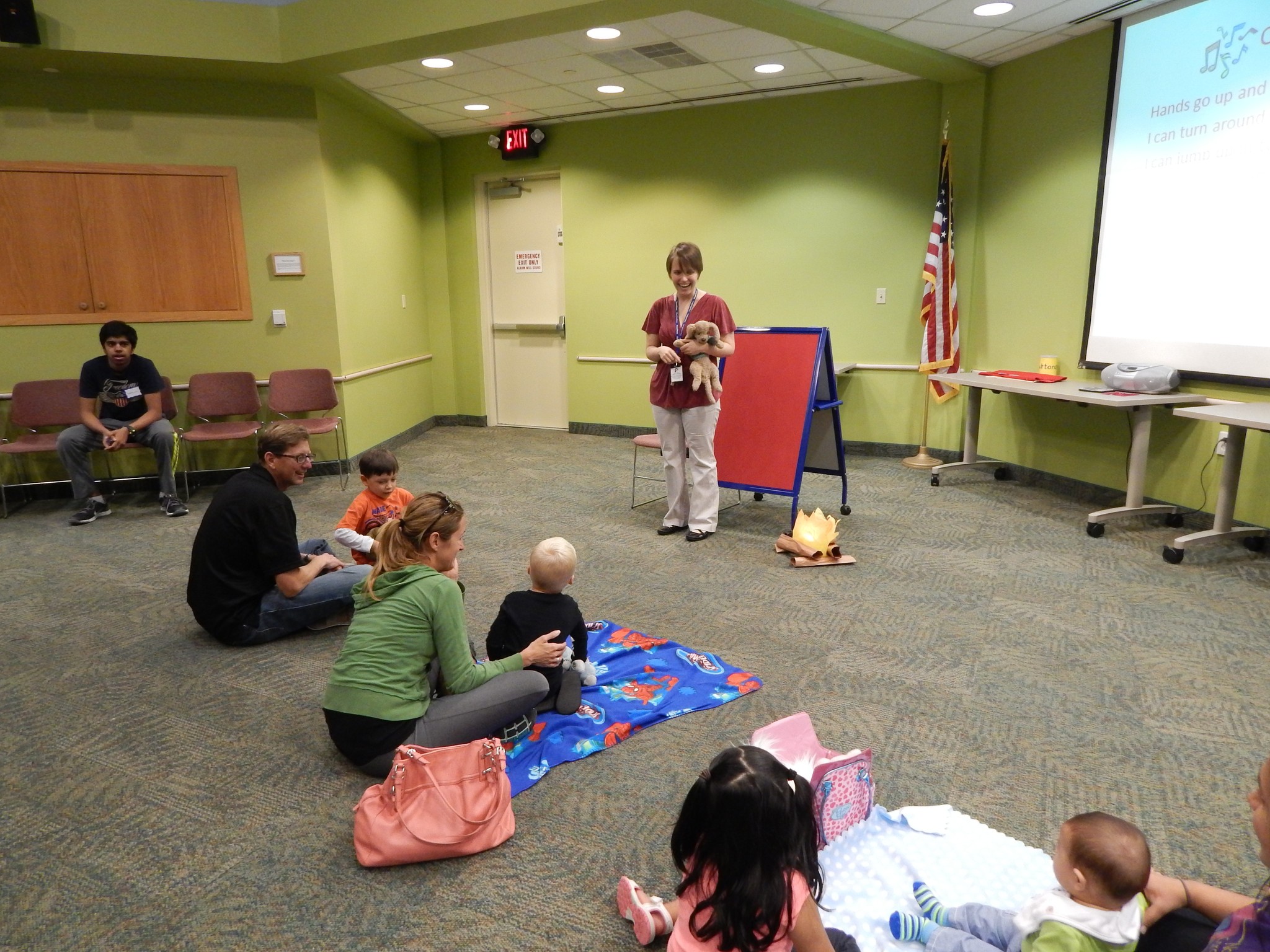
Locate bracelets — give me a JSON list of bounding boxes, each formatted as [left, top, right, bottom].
[[304, 553, 314, 563]]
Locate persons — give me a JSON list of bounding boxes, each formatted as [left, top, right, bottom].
[[322, 491, 568, 781], [617, 745, 861, 952], [186, 422, 375, 646], [1135, 756, 1270, 952], [890, 810, 1151, 952], [56, 320, 188, 524], [641, 242, 737, 541], [486, 537, 588, 713], [334, 448, 415, 568]]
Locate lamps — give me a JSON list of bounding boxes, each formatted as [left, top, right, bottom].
[[487, 124, 545, 160]]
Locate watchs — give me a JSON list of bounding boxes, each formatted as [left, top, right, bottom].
[[123, 425, 136, 438]]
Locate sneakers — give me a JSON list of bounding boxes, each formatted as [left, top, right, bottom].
[[158, 495, 189, 517], [68, 499, 112, 523]]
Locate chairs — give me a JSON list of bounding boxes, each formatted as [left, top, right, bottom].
[[269, 368, 350, 491], [0, 379, 115, 519], [630, 433, 741, 512], [103, 376, 200, 497], [181, 371, 267, 503]]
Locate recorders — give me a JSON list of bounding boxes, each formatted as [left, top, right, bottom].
[[1100, 362, 1181, 395]]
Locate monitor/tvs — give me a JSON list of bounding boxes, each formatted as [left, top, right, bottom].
[[0, 0, 42, 45]]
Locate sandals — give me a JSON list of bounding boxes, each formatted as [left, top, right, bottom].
[[617, 876, 674, 946]]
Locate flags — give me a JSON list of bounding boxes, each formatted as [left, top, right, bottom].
[[920, 138, 960, 402]]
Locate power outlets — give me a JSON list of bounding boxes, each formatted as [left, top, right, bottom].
[[1217, 431, 1228, 455]]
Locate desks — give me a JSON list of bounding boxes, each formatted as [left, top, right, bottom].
[[1161, 402, 1270, 564], [651, 358, 857, 389], [929, 373, 1208, 541]]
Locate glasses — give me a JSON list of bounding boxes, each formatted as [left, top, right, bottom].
[[421, 491, 463, 542], [273, 454, 316, 463]]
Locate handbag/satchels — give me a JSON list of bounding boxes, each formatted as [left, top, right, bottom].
[[751, 711, 875, 850], [352, 738, 515, 867]]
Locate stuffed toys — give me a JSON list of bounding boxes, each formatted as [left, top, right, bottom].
[[673, 320, 724, 404], [562, 645, 597, 686]]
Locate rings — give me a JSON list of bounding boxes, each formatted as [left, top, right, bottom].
[[557, 657, 559, 662]]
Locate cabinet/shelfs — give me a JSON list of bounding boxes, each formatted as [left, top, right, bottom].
[[0, 161, 254, 326]]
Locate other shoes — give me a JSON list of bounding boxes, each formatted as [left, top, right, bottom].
[[686, 529, 714, 541], [306, 608, 355, 630], [657, 525, 688, 535], [492, 709, 537, 745], [556, 671, 581, 715], [535, 695, 555, 711]]
